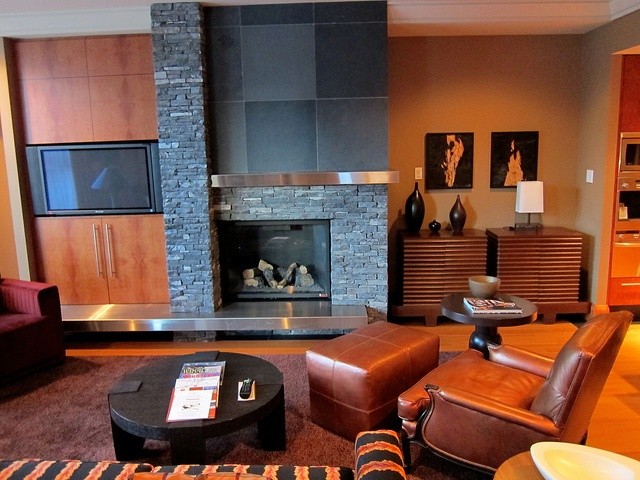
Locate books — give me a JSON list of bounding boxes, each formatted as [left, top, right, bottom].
[[166, 360, 226, 423], [463, 295, 523, 315]]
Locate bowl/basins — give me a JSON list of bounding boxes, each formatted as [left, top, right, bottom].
[[468, 275, 500, 299]]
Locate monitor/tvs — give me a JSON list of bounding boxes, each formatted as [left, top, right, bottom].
[[25, 143, 163, 215]]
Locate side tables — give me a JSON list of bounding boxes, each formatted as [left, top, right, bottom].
[[440, 291, 538, 361]]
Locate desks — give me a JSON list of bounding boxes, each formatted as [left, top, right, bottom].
[[486, 226, 584, 305], [494, 449, 543, 480], [397, 227, 488, 306]]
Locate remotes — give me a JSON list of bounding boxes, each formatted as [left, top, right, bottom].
[[239, 378, 253, 399]]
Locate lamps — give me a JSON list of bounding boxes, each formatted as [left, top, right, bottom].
[[514, 180, 544, 229]]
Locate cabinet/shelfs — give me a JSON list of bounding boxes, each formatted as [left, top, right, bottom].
[[29, 213, 169, 306], [607, 232, 640, 307], [619, 53, 640, 133]]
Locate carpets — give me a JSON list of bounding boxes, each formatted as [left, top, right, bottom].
[[0, 350, 490, 461]]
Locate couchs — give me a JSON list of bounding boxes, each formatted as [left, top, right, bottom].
[[396, 309, 634, 472], [304, 320, 440, 441], [0, 427, 407, 480], [0, 274, 65, 376]]
